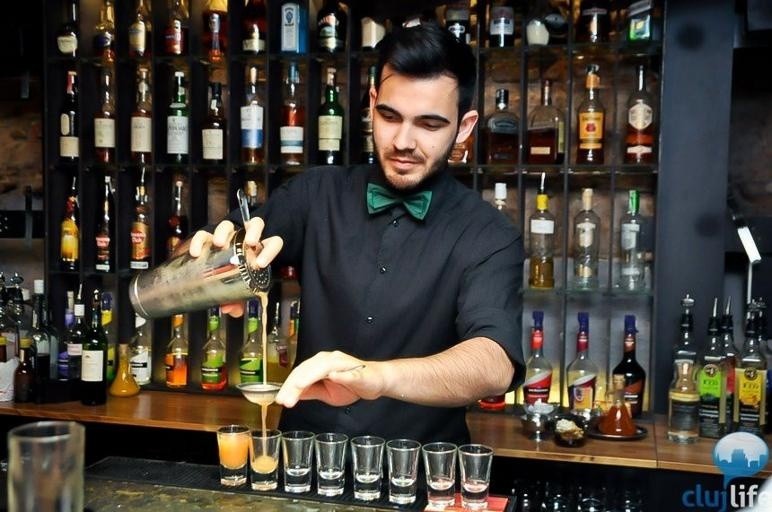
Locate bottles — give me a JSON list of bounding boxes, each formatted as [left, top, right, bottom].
[[0, 0, 772, 448]]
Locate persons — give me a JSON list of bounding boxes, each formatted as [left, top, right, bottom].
[[169, 24, 526, 448]]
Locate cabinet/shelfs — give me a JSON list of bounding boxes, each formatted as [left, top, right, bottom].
[[46, 2, 664, 408]]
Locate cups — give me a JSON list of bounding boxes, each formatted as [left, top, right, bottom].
[[217, 426, 496, 511], [510, 477, 646, 512], [7, 420, 86, 511]]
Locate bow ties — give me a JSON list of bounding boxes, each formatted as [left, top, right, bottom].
[[364, 182, 434, 221]]
